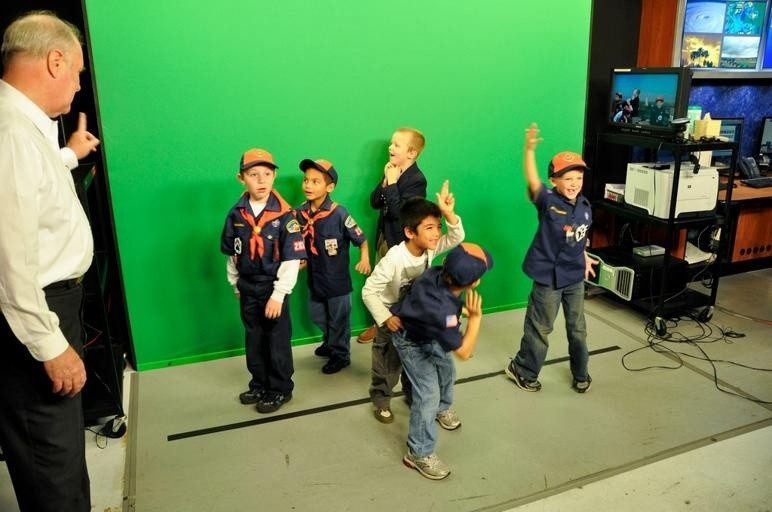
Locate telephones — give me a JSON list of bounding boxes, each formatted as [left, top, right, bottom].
[[738, 157, 761, 179]]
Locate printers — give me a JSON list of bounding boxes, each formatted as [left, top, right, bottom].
[[624, 160, 720, 220]]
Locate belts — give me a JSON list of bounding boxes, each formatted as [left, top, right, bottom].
[[41, 275, 86, 291]]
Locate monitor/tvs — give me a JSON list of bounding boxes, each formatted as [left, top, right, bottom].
[[701, 117, 744, 170], [606, 65, 692, 138], [757, 117, 772, 163]]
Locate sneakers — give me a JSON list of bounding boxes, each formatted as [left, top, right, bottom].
[[323, 357, 352, 374], [433, 409, 461, 431], [571, 373, 593, 394], [314, 347, 332, 357], [373, 398, 394, 424], [256, 390, 292, 413], [356, 327, 377, 344], [505, 360, 543, 393], [402, 449, 451, 482], [239, 387, 265, 404]]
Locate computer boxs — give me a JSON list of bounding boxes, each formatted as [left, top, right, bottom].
[[585, 244, 689, 304]]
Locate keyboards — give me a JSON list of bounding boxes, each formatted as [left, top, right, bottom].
[[740, 177, 772, 188], [718, 182, 737, 190]]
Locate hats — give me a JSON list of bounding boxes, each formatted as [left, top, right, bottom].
[[443, 242, 495, 285], [547, 151, 592, 178], [298, 156, 339, 183], [655, 96, 665, 101], [615, 92, 623, 98], [238, 148, 279, 172]]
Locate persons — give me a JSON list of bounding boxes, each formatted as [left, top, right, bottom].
[[390, 242, 494, 481], [358, 179, 466, 421], [221, 149, 309, 413], [614, 89, 669, 125], [0, 12, 100, 512], [358, 128, 426, 343], [292, 159, 370, 375], [506, 123, 598, 394]]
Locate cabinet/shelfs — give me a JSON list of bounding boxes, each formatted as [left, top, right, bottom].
[[593, 118, 742, 317], [636, 0, 771, 278]]
